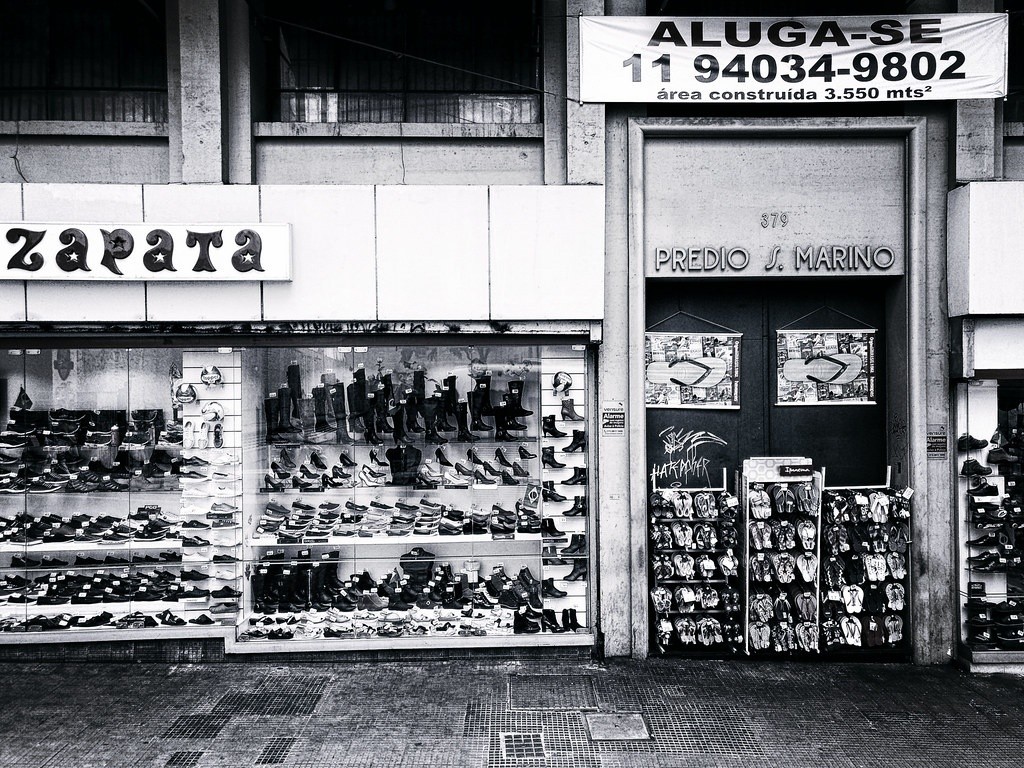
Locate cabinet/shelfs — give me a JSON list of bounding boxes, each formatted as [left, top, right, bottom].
[[0, 333, 1024, 665]]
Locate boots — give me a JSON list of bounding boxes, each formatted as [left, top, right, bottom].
[[541, 398, 589, 635], [263, 362, 535, 446]]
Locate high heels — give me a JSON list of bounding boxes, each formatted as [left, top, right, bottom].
[[257, 448, 540, 488]]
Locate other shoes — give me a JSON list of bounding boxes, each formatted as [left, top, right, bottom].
[[959, 431, 1024, 652], [248, 500, 544, 639], [1, 405, 243, 633]]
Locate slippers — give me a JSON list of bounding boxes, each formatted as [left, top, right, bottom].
[[647, 356, 727, 389], [783, 353, 865, 386], [649, 483, 911, 653]]
